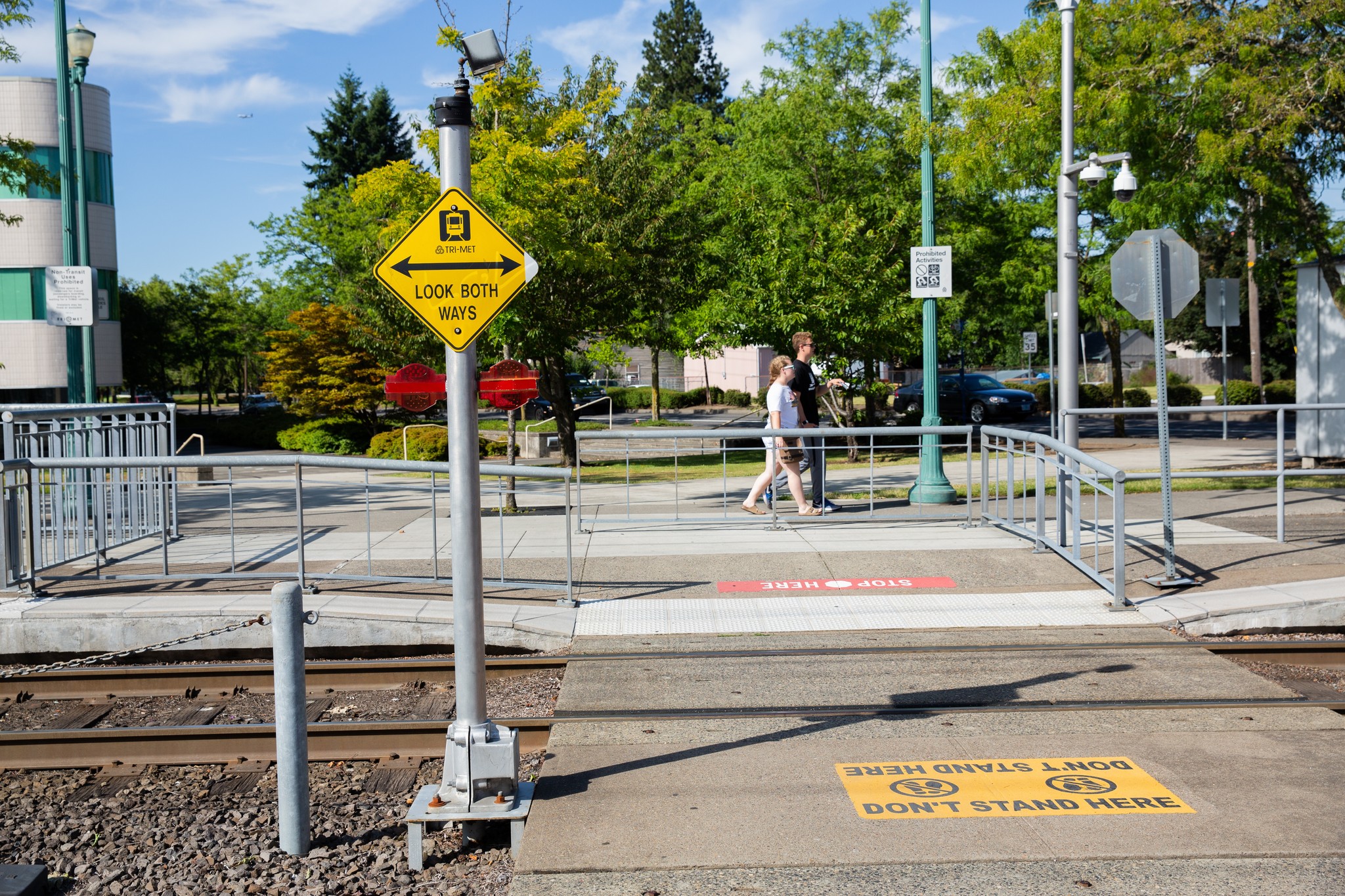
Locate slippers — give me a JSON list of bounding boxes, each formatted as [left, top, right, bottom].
[[798, 506, 823, 516], [741, 505, 766, 514]]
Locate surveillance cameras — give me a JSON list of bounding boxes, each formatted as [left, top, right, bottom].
[[1079, 164, 1107, 188], [1112, 162, 1138, 203]]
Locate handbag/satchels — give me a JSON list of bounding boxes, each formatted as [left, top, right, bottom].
[[778, 437, 805, 464]]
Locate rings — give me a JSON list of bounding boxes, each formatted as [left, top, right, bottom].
[[776, 443, 779, 445]]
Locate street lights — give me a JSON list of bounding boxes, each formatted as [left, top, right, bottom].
[[64, 19, 99, 407]]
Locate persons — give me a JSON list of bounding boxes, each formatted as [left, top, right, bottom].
[[740, 354, 823, 516], [762, 331, 846, 511]]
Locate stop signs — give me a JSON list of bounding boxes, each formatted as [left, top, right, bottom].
[[715, 579, 960, 589]]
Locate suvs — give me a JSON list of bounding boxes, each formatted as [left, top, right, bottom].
[[506, 373, 652, 420]]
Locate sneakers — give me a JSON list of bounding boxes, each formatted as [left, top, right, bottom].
[[812, 497, 842, 511], [762, 482, 778, 510]]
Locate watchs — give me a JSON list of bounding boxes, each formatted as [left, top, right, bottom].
[[801, 420, 809, 426]]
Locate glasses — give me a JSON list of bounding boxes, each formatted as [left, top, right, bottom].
[[799, 342, 815, 348], [779, 364, 795, 372]]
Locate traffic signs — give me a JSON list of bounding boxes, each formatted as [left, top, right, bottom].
[[372, 186, 540, 350]]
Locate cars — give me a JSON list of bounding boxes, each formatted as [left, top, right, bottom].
[[243, 394, 282, 414], [131, 392, 175, 403], [893, 374, 1040, 423]]
